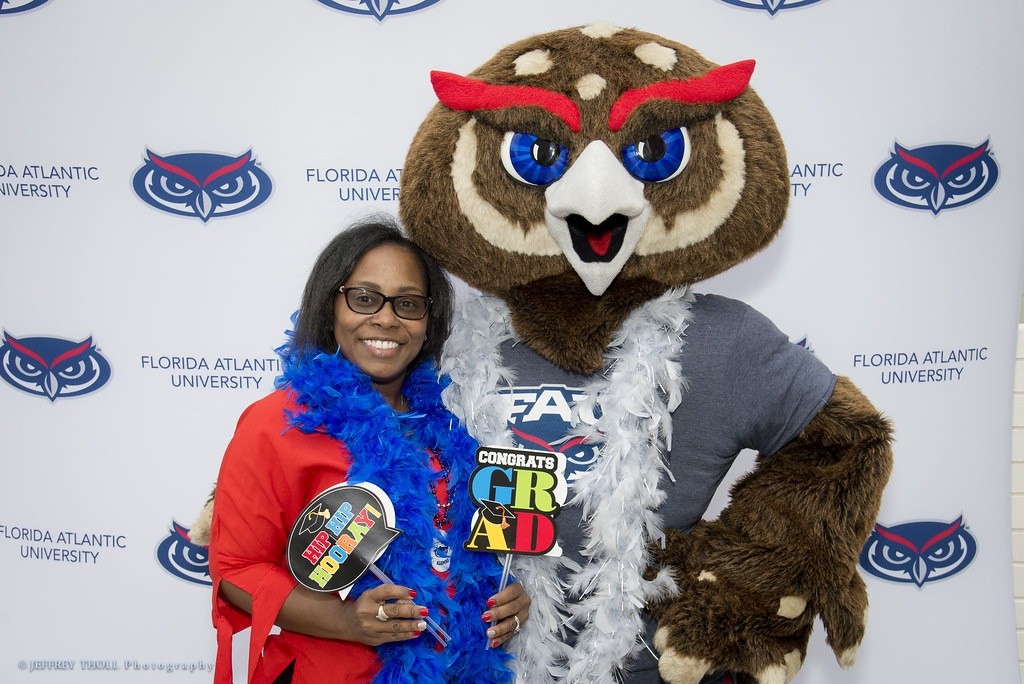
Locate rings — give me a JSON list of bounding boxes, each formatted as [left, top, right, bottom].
[[375, 604, 389, 622], [513, 616, 522, 634]]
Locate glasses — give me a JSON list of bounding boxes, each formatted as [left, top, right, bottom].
[[338, 286, 433, 320]]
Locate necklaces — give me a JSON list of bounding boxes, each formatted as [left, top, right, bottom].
[[274, 309, 518, 684], [439, 280, 697, 684]]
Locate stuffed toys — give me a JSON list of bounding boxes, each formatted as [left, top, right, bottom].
[[188, 26, 894, 684]]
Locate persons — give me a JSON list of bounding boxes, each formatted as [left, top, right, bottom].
[[207, 221, 533, 684]]
